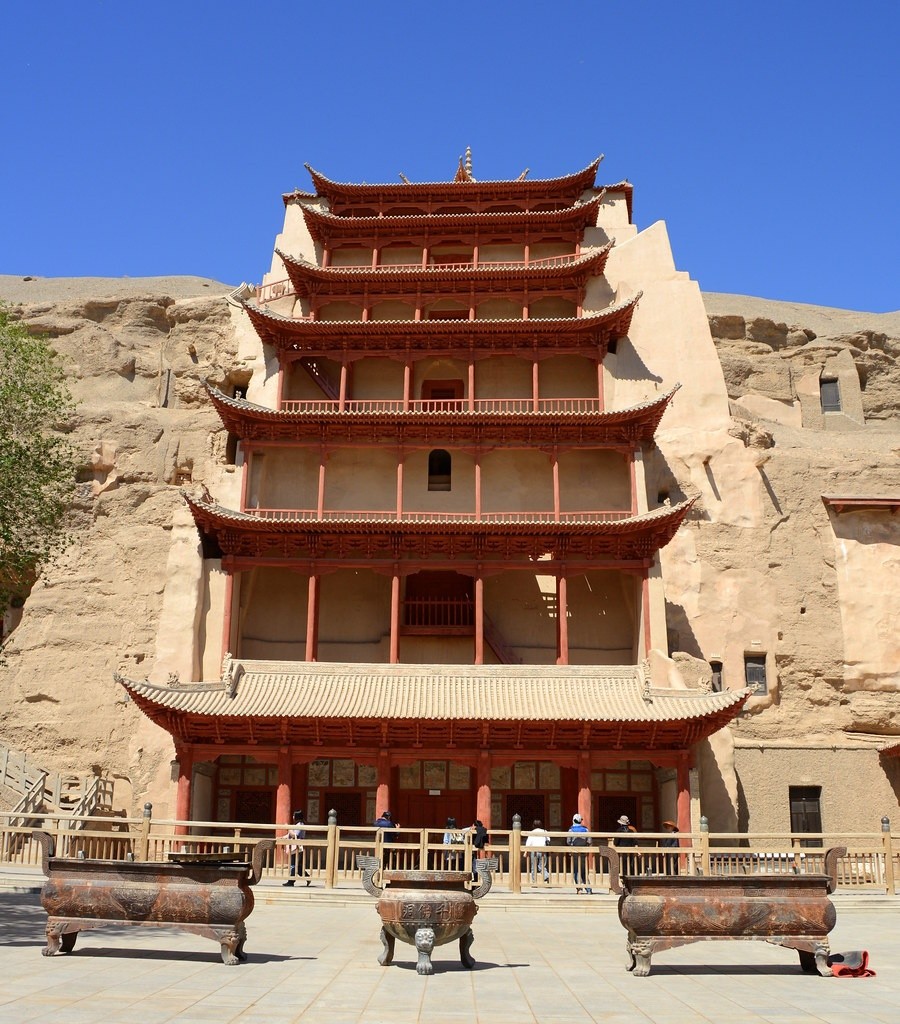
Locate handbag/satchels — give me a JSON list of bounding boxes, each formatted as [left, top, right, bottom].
[[544, 829, 551, 857], [285, 832, 304, 855]]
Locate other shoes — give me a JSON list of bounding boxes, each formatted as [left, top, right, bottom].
[[283, 882, 294, 886], [578, 890, 581, 894], [307, 880, 311, 886], [588, 891, 592, 895]]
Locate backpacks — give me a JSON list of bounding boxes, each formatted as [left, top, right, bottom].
[[450, 833, 464, 854]]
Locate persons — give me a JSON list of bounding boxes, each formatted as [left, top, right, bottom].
[[613, 815, 639, 877], [442, 816, 462, 871], [660, 820, 679, 875], [276, 809, 312, 887], [461, 820, 483, 887], [567, 813, 592, 894], [523, 819, 551, 888], [372, 811, 400, 871]]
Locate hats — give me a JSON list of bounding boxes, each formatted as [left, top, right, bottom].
[[663, 821, 679, 832], [572, 814, 583, 826], [616, 815, 631, 825], [628, 826, 637, 833]]
